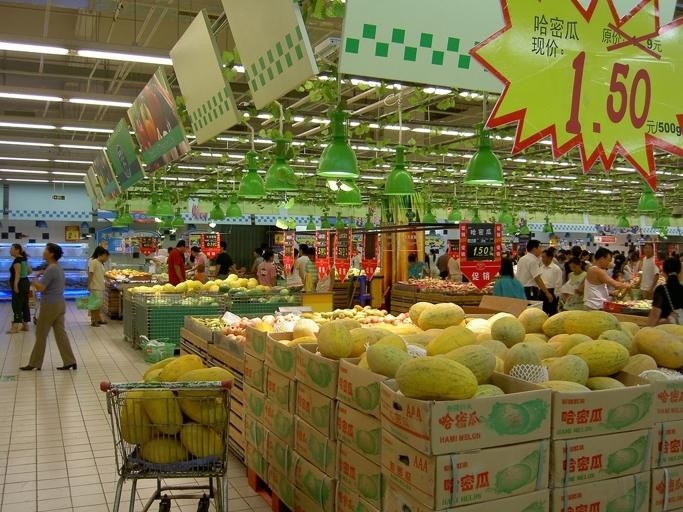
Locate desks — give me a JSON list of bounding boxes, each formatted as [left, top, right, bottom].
[[101, 275, 648, 463]]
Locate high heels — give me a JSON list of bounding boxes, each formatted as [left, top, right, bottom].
[[19, 366, 40, 370], [57, 363, 76, 370]]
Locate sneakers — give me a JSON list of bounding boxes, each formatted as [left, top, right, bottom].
[[91, 320, 108, 327]]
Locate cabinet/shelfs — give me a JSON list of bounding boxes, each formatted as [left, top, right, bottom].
[[0, 243, 90, 300]]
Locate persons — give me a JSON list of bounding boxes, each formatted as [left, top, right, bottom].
[[406, 247, 462, 281], [492, 240, 683, 325], [5, 244, 110, 371], [166, 241, 209, 286], [215, 241, 238, 280], [251, 242, 317, 290]]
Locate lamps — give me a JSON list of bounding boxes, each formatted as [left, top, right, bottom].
[[80, 222, 89, 228], [0, 34, 671, 229], [39, 220, 47, 227]]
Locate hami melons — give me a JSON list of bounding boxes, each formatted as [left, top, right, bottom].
[[277, 300, 683, 402], [128, 274, 271, 294], [119, 353, 236, 462]]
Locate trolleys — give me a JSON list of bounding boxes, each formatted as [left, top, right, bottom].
[[27, 285, 41, 324], [101, 379, 232, 512]]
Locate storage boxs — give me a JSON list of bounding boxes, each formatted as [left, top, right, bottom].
[[245, 327, 683, 512]]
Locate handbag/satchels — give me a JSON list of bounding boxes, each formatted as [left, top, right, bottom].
[[668, 308, 683, 325], [87, 294, 102, 310]]
